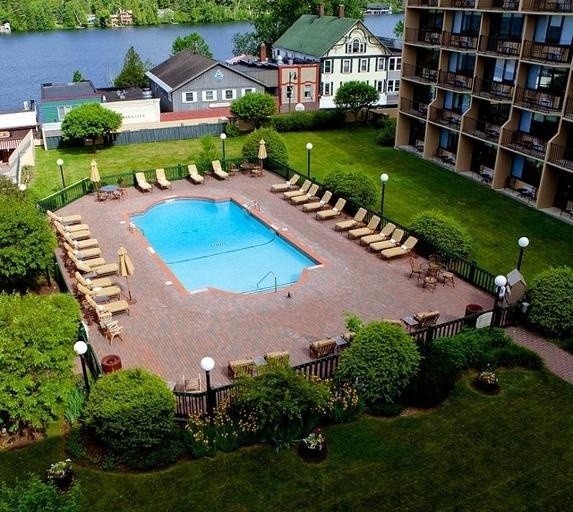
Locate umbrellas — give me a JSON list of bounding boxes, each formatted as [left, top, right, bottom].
[[88, 158, 100, 200], [257, 137, 267, 174], [115, 246, 136, 302]]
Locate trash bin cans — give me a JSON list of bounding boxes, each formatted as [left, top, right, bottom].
[[101, 355, 122, 374], [465, 304, 482, 326]]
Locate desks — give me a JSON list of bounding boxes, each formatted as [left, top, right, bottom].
[[399, 316, 419, 332]]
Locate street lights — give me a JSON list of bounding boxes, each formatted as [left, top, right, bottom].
[[55, 159, 68, 201], [287, 71, 296, 113], [379, 173, 389, 215], [218, 132, 227, 170], [305, 142, 314, 179], [72, 339, 90, 399], [488, 275, 507, 334], [198, 356, 219, 424], [18, 183, 26, 198], [515, 235, 531, 269]]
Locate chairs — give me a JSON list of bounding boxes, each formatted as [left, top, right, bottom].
[[308, 331, 356, 359], [226, 347, 289, 382], [412, 309, 440, 330], [182, 373, 201, 396], [270, 173, 459, 294], [95, 168, 170, 204], [45, 210, 129, 345], [186, 157, 260, 186]]
